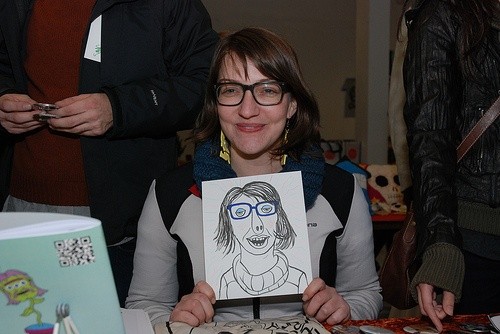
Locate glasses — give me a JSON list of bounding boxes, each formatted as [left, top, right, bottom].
[[214, 80, 290, 106]]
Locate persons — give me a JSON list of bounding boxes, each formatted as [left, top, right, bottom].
[[0, 0, 222, 308], [125, 26, 383, 327], [396, 0, 500, 332]]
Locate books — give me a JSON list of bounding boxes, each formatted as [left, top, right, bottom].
[[0, 212, 126, 334]]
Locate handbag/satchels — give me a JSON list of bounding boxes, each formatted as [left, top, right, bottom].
[[378, 227, 420, 310]]
